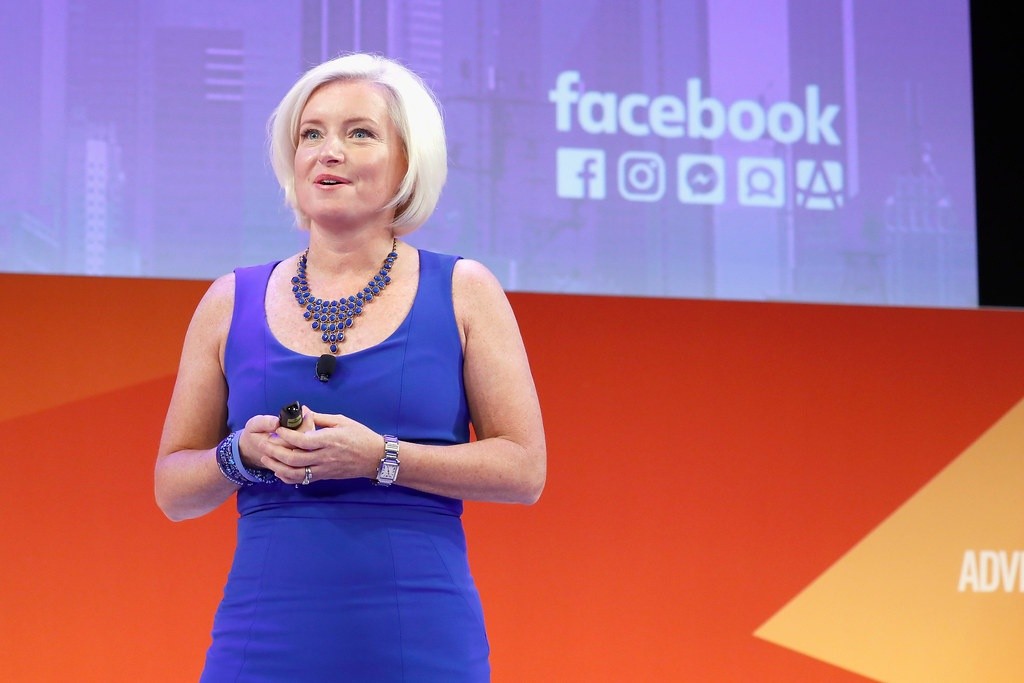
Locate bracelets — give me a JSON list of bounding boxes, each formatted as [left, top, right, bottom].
[[216, 428, 277, 486]]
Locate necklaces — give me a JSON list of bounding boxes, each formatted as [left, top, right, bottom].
[[292, 235, 398, 353]]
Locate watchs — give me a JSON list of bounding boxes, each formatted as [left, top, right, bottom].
[[369, 434, 400, 488]]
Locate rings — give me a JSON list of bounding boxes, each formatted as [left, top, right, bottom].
[[302, 466, 312, 484], [294, 483, 299, 488]]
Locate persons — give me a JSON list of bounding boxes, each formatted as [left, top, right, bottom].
[[154, 53, 548, 683]]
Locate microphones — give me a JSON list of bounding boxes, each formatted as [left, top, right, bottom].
[[315, 354, 337, 381]]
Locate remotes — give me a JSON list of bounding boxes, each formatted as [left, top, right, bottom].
[[278, 400, 303, 430]]
